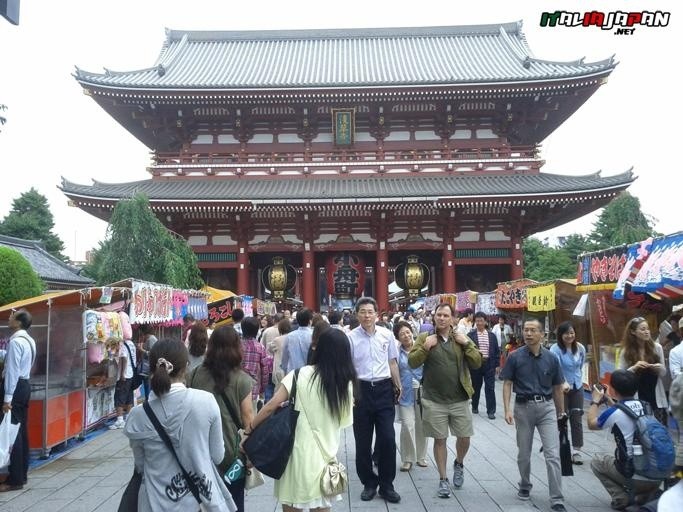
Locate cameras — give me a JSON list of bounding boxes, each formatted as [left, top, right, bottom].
[[592, 382, 607, 395]]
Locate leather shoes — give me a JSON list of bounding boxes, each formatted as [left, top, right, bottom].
[[1, 483, 21, 492], [418, 461, 427, 466], [489, 414, 495, 419], [361, 488, 377, 500], [401, 463, 411, 471], [379, 487, 401, 502], [472, 409, 478, 413]]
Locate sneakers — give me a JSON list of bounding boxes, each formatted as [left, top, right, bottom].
[[453, 459, 464, 486], [518, 490, 529, 500], [552, 504, 567, 512], [573, 450, 583, 464], [609, 495, 635, 510], [126, 414, 128, 420], [109, 421, 125, 429], [437, 479, 450, 498], [633, 494, 658, 504]]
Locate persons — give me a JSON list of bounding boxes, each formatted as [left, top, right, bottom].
[[497, 319, 570, 512], [265, 320, 292, 391], [550, 322, 586, 466], [103, 338, 120, 364], [124, 335, 239, 512], [185, 323, 208, 372], [407, 302, 483, 498], [231, 308, 245, 334], [491, 315, 514, 377], [239, 315, 270, 418], [617, 316, 670, 428], [239, 327, 358, 512], [586, 367, 677, 510], [253, 304, 473, 334], [108, 338, 137, 431], [392, 320, 428, 473], [0, 309, 38, 492], [184, 324, 257, 511], [466, 311, 499, 420], [136, 323, 159, 404], [346, 297, 403, 504], [280, 309, 314, 376], [669, 315, 683, 426], [182, 313, 196, 344]]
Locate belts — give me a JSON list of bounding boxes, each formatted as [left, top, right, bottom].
[[517, 393, 552, 402], [483, 358, 489, 362], [361, 378, 389, 386]]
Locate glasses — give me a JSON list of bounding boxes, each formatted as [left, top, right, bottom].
[[632, 316, 646, 322]]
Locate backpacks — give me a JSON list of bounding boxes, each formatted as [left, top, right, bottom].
[[611, 399, 675, 480]]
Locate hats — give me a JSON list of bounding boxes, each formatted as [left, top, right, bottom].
[[417, 308, 424, 314]]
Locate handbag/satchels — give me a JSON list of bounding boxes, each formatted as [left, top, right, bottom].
[[142, 399, 206, 512], [557, 413, 574, 477], [1, 334, 37, 380], [393, 383, 400, 405], [290, 370, 349, 496], [211, 369, 266, 489], [137, 350, 151, 375], [0, 409, 22, 470], [117, 469, 143, 512], [124, 342, 142, 392], [242, 369, 301, 480]]
